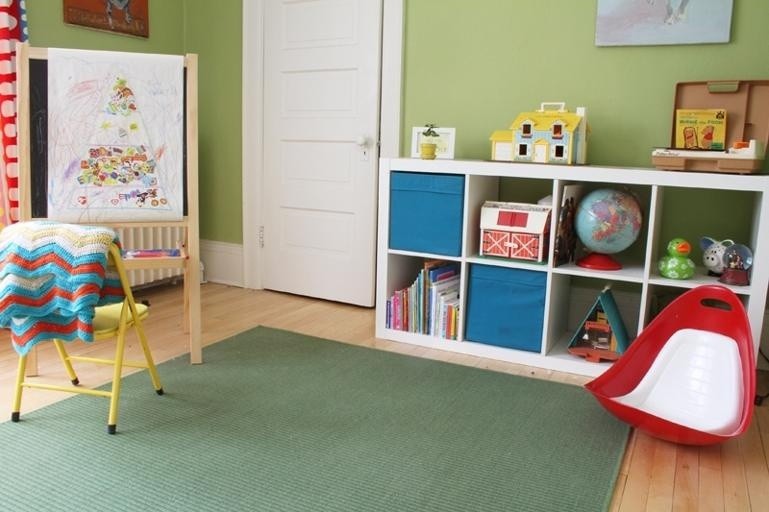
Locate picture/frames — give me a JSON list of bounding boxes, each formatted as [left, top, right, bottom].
[[412, 127, 456, 160]]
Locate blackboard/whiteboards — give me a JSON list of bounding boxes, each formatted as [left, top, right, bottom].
[[16, 47, 199, 228]]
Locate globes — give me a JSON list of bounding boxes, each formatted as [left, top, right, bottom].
[[573, 184, 646, 270]]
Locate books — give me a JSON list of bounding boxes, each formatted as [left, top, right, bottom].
[[384, 260, 461, 343], [551, 183, 580, 269]]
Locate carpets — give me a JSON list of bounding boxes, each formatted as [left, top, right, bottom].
[[1, 324, 634, 511]]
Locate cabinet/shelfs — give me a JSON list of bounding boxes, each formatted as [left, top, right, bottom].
[[374, 157, 769, 378]]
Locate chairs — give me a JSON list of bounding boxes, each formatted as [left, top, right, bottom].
[[10, 243, 163, 434], [583, 285, 756, 449]]
